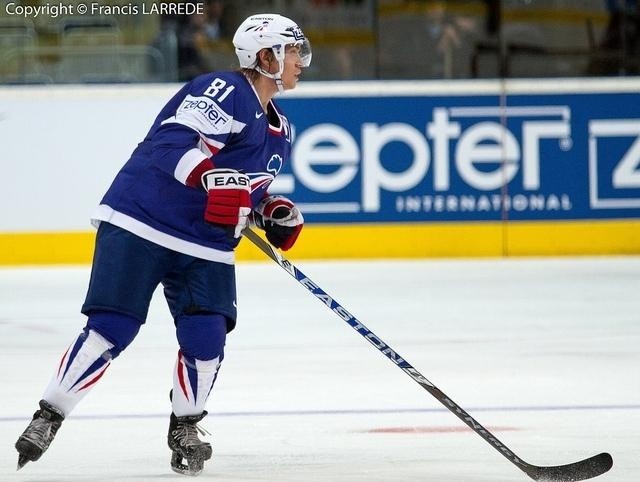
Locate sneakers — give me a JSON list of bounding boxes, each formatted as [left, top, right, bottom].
[[167, 409, 213, 463], [15, 399, 65, 463]]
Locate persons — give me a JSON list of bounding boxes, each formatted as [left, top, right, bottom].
[[15, 14, 311, 466], [145, 0, 639, 82]]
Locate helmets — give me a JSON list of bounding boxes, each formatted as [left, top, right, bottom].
[[232, 14, 313, 69]]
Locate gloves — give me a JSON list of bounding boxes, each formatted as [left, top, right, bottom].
[[201, 167, 251, 241], [252, 193, 306, 253]]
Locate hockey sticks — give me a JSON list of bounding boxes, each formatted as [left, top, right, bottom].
[[240, 228, 613, 481]]
